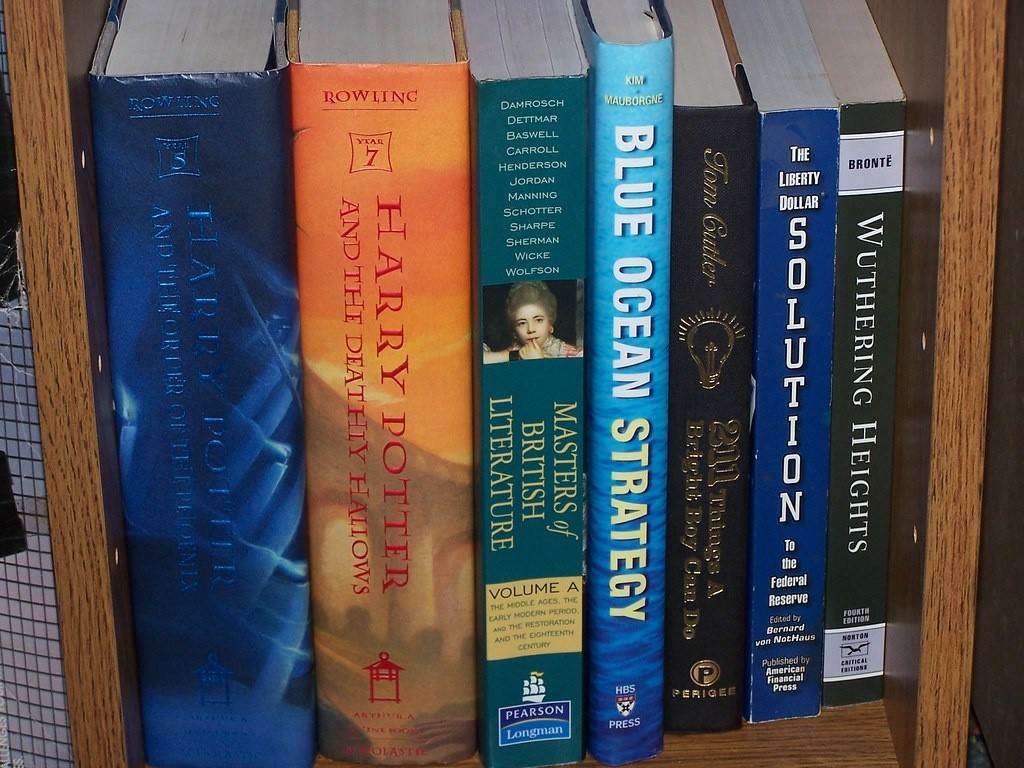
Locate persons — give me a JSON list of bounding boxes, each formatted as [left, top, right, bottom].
[[482, 281, 582, 363]]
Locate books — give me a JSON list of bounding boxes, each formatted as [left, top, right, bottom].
[[85, 0, 909, 768]]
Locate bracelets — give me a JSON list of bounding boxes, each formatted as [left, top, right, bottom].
[[509, 350, 519, 362]]
